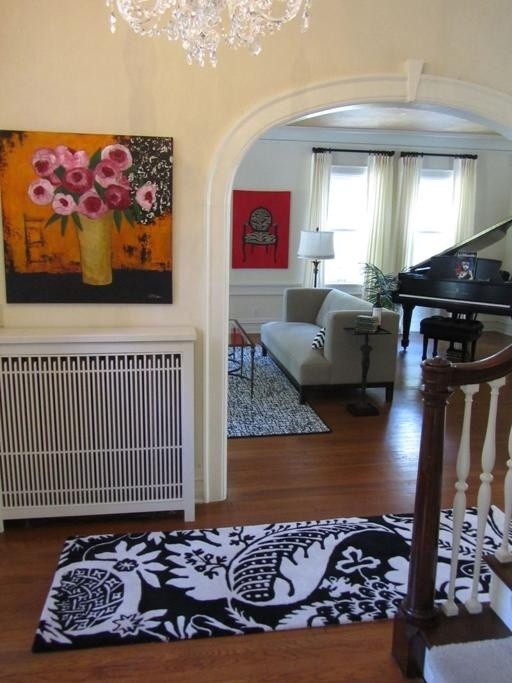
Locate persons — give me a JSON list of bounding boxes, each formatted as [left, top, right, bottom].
[[457, 257, 473, 278]]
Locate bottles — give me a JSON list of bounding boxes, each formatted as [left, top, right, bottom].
[[371, 293, 383, 330]]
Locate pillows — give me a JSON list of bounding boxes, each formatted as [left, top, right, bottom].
[[311, 327, 325, 350]]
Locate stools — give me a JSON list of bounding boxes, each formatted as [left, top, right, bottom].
[[420, 315, 483, 361]]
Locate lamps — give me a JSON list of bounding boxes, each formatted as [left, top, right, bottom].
[[103, 0, 312, 71], [296, 227, 335, 288]]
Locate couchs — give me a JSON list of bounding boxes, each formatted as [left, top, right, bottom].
[[259, 288, 400, 404]]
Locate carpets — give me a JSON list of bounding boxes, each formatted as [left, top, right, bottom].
[[228, 344, 332, 439], [31, 505, 512, 653]]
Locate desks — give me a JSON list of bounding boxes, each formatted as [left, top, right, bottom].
[[344, 326, 392, 417]]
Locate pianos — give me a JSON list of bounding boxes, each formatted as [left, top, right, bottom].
[[391, 218, 512, 351]]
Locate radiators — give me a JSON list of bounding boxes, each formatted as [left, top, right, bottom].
[[0, 325, 198, 532]]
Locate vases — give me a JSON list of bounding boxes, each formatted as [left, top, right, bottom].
[[75, 208, 114, 286]]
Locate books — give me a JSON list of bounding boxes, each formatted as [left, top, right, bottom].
[[355, 314, 378, 333]]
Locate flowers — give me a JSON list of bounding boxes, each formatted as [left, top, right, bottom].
[[27, 142, 158, 236]]
